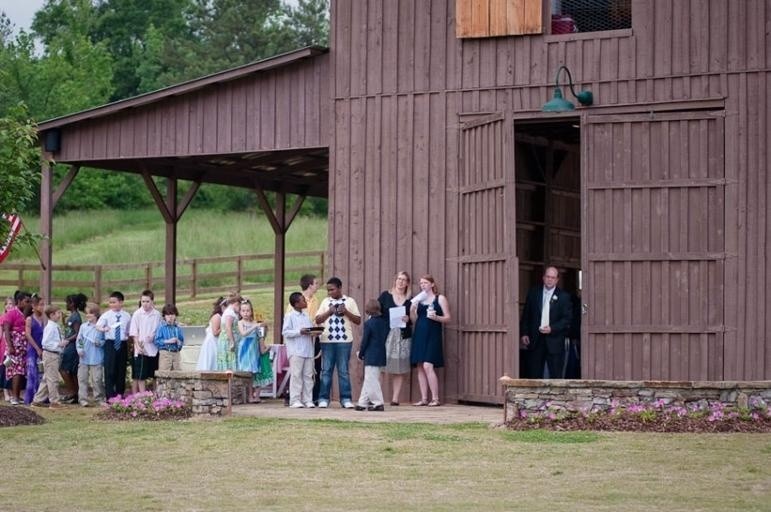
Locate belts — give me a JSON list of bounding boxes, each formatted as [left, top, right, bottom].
[[42, 348, 63, 356], [159, 347, 182, 353]]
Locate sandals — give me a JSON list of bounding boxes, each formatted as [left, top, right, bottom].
[[411, 398, 442, 407], [390, 399, 400, 407]]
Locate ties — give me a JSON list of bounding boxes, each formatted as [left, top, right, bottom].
[[112, 314, 122, 352], [540, 291, 550, 328]]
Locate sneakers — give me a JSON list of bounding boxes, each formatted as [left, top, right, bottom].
[[339, 400, 355, 409], [318, 399, 330, 408], [288, 398, 316, 409], [4, 395, 109, 411]]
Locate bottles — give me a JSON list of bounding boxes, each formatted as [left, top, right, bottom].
[[427, 301, 435, 318]]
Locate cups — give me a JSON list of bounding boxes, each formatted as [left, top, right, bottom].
[[256, 327, 267, 338]]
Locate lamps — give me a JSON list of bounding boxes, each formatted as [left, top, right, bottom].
[[541, 64, 595, 114]]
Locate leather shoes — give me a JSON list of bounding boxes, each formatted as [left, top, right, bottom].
[[368, 404, 385, 411], [355, 405, 366, 411]]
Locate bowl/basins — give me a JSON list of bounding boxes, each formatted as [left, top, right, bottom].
[[307, 327, 325, 336]]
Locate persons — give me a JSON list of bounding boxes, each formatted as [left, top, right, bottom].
[[521, 266, 573, 379], [409, 274, 451, 407], [2, 284, 272, 409], [356, 296, 387, 411], [286, 273, 321, 406], [376, 271, 413, 406], [282, 291, 317, 409], [315, 277, 361, 408]]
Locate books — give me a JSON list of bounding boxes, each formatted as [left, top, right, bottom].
[[389, 305, 407, 328]]
